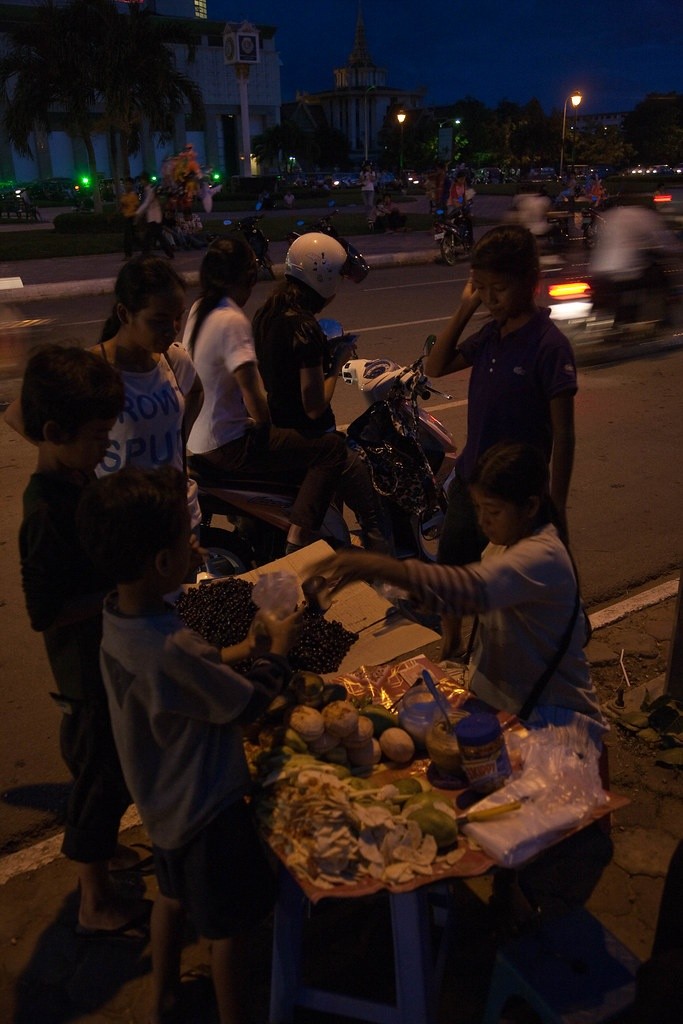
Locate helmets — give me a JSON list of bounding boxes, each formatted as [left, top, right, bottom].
[[283, 231, 348, 300]]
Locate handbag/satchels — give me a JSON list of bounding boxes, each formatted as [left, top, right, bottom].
[[344, 361, 439, 515]]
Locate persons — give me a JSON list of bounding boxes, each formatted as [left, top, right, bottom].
[[432, 163, 468, 221], [251, 229, 400, 563], [72, 459, 285, 1024], [15, 351, 209, 941], [254, 187, 296, 209], [118, 174, 215, 262], [419, 224, 581, 661], [367, 195, 413, 234], [312, 445, 601, 935], [588, 179, 683, 344], [4, 255, 217, 585], [179, 231, 345, 551], [359, 159, 376, 216]]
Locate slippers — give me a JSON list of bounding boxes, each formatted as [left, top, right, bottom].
[[96, 841, 158, 875], [76, 900, 150, 944]]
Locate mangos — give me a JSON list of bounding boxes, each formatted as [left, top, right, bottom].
[[358, 705, 399, 733], [324, 764, 459, 849]]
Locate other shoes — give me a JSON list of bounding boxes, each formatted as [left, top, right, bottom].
[[150, 963, 213, 1024]]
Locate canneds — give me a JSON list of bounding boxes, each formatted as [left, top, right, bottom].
[[455, 713, 513, 795]]
